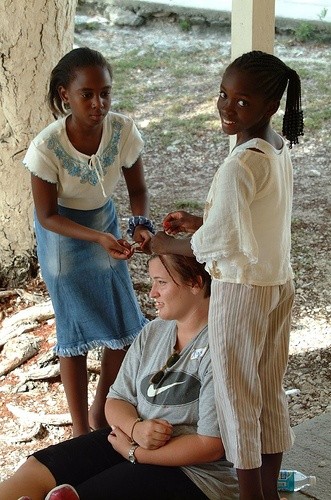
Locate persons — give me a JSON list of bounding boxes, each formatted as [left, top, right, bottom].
[[0, 247, 242, 500], [22, 48, 155, 438], [150, 52, 305, 500]]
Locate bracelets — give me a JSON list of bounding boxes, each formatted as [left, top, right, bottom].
[[126, 214, 156, 239], [130, 419, 143, 442]]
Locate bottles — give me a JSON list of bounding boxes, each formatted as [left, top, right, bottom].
[[276, 470, 316, 492]]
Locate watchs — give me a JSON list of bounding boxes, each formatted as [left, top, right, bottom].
[[129, 444, 137, 464]]
